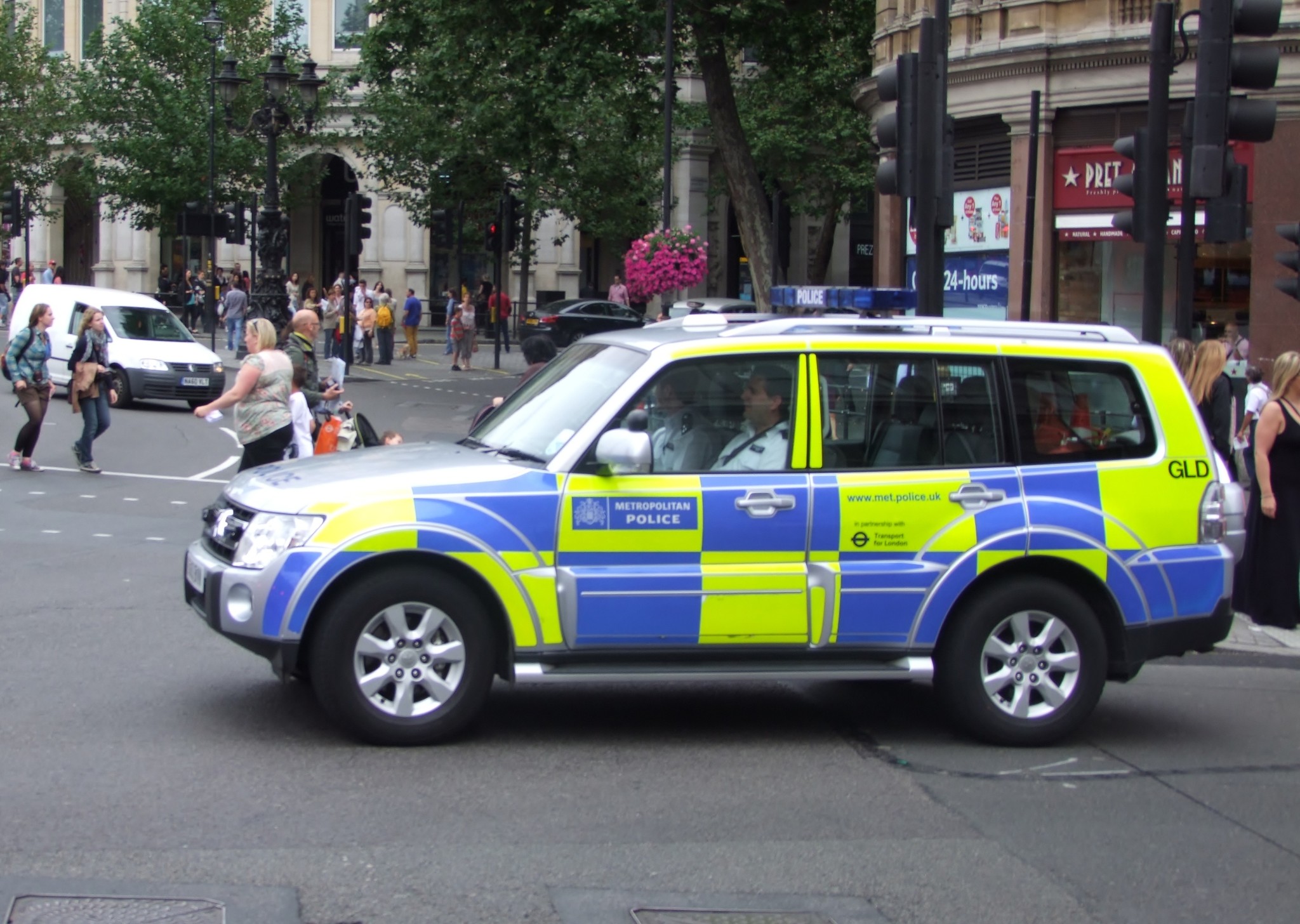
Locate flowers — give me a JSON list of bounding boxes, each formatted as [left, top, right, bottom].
[[623, 226, 708, 301]]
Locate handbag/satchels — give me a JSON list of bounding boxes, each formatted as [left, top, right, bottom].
[[314, 415, 342, 454]]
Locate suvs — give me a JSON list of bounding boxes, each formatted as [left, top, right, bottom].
[[167, 284, 1254, 740]]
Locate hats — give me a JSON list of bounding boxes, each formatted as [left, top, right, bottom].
[[49, 260, 56, 264]]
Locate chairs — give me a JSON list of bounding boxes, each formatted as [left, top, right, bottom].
[[868, 376, 938, 468], [944, 375, 999, 465]]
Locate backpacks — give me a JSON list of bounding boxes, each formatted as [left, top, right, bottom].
[[0, 326, 34, 380]]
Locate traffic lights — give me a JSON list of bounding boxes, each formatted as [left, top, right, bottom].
[[483, 223, 500, 254], [429, 207, 453, 250], [223, 200, 245, 245], [872, 50, 915, 196], [1111, 129, 1145, 243], [349, 194, 372, 253], [1274, 221, 1299, 303], [1186, 1, 1284, 201], [500, 195, 526, 251], [186, 199, 202, 218], [0, 187, 23, 235]]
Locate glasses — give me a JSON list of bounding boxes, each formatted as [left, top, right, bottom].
[[251, 318, 258, 332], [303, 322, 322, 328]]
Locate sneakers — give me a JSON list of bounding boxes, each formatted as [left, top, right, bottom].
[[21, 460, 44, 472], [8, 450, 21, 471]]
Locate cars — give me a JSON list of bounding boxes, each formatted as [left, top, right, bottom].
[[8, 283, 226, 412], [517, 299, 659, 349]]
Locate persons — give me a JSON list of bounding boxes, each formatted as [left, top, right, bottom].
[[0, 258, 65, 327], [1232, 350, 1300, 629], [1168, 324, 1270, 490], [287, 273, 322, 315], [6, 303, 55, 472], [67, 308, 118, 472], [443, 273, 511, 371], [492, 334, 557, 408], [710, 365, 791, 472], [381, 431, 403, 445], [607, 275, 630, 307], [194, 309, 353, 474], [401, 289, 422, 358], [822, 360, 854, 441], [648, 374, 715, 472], [159, 263, 250, 350], [322, 270, 397, 364]]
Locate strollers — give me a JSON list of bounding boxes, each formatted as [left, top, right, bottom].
[[307, 375, 385, 449]]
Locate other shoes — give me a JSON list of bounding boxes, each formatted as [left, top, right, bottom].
[[0, 319, 7, 327], [81, 461, 100, 472], [355, 359, 364, 364], [374, 361, 390, 365], [192, 329, 199, 333], [411, 354, 417, 358], [361, 362, 371, 366], [462, 365, 473, 370], [73, 445, 84, 466], [452, 365, 461, 371]]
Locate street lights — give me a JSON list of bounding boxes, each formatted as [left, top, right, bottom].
[[216, 51, 329, 356], [202, 20, 222, 354]]
